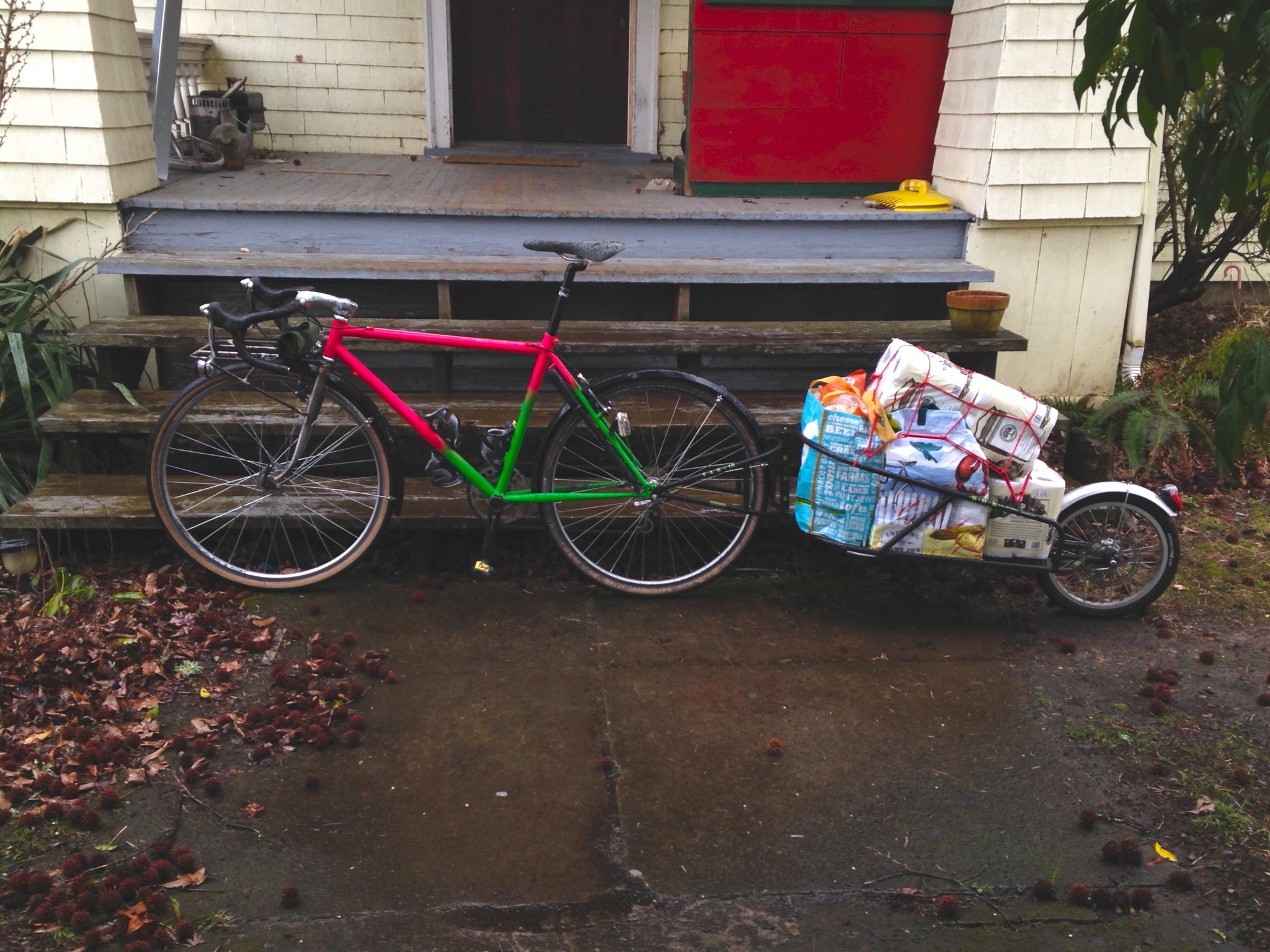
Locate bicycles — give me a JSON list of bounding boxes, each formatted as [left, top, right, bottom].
[[145, 238, 772, 600]]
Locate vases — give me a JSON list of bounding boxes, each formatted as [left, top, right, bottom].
[[948, 288, 1011, 336]]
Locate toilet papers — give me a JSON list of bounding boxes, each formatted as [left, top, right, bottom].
[[982, 459, 1066, 560]]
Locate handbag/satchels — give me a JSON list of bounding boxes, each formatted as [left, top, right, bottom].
[[870, 402, 988, 562], [794, 375, 897, 547]]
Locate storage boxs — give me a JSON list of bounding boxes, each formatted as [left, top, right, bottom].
[[987, 458, 1066, 559]]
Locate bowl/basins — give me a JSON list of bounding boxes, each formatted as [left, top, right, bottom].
[[946, 289, 1011, 338]]
